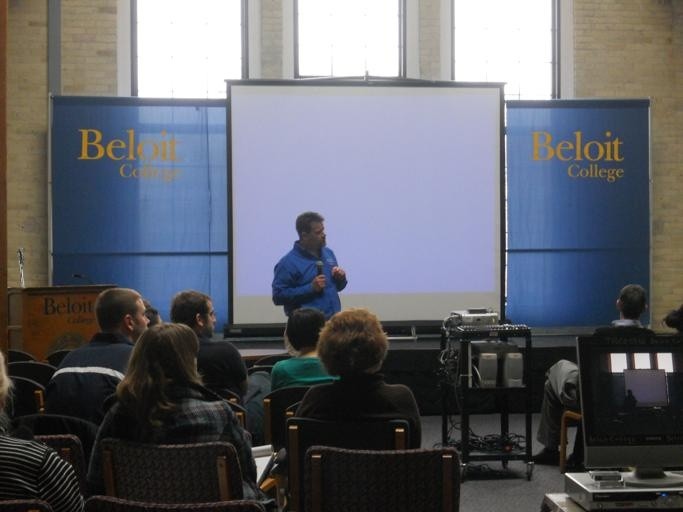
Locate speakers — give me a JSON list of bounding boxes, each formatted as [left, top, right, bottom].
[[478, 353, 498, 388], [502, 352, 523, 388]]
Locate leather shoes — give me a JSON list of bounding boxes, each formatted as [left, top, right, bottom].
[[532, 449, 560, 466], [568, 453, 582, 464]]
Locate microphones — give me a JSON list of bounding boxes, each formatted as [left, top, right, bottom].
[[315, 261, 325, 276], [16, 247, 25, 268]]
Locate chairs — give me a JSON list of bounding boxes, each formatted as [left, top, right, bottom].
[[560, 410, 583, 475], [305, 445, 460, 512], [98, 438, 279, 512], [208, 355, 314, 476], [83, 495, 265, 512], [286, 416, 410, 512], [0, 350, 98, 512]]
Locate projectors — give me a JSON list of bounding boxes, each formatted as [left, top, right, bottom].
[[452, 309, 499, 325]]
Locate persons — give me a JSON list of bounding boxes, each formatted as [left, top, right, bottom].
[[170, 289, 246, 401], [270, 211, 348, 320], [623, 389, 637, 409], [45, 286, 152, 412], [85, 322, 257, 499], [0, 351, 84, 511], [294, 304, 423, 451], [530, 283, 656, 467], [268, 304, 342, 393], [140, 298, 164, 328]]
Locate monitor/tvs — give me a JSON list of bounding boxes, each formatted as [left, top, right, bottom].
[[575, 333, 682, 487]]
